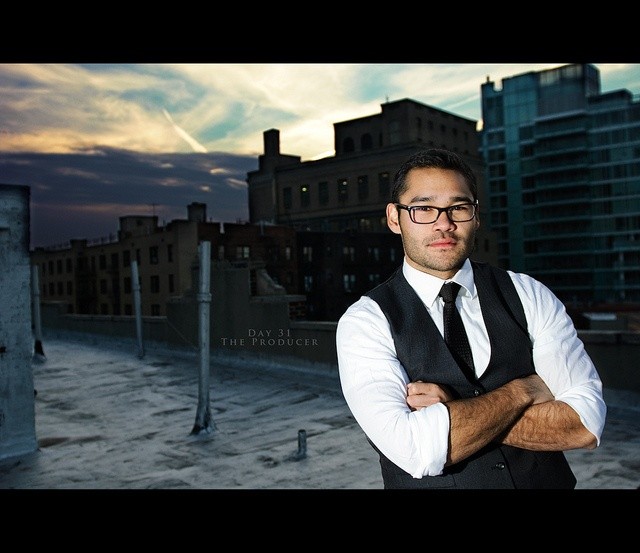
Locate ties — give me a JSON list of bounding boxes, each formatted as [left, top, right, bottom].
[[438, 282, 475, 383]]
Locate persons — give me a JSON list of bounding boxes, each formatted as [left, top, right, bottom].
[[334, 147, 607, 489]]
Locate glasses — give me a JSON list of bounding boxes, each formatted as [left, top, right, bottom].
[[395, 199, 479, 224]]
